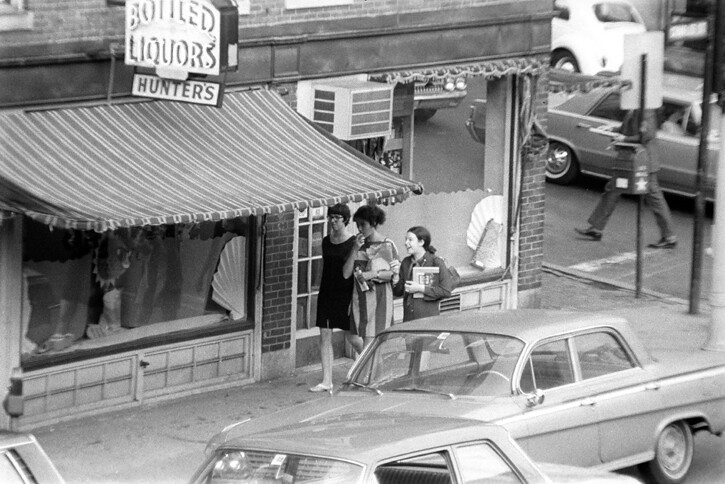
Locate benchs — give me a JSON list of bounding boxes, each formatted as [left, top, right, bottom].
[[524, 360, 628, 382]]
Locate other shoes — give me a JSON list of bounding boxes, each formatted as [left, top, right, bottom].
[[309, 384, 334, 391], [574, 225, 603, 240], [649, 235, 677, 250]]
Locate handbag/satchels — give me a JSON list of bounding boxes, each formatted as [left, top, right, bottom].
[[432, 257, 460, 291]]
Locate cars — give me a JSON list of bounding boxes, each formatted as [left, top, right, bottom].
[[550, 0, 647, 76], [463, 84, 722, 206], [368, 69, 467, 125], [203, 307, 725, 484], [186, 411, 643, 484]]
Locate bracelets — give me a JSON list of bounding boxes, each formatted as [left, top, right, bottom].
[[377, 271, 379, 279]]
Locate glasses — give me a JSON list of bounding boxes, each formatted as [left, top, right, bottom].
[[327, 215, 343, 222]]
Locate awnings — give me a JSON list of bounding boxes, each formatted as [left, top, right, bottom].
[[0, 88, 423, 235]]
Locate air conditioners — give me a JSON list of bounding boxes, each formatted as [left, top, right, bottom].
[[310, 81, 395, 140]]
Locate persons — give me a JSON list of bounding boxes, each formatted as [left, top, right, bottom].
[[309, 203, 452, 393], [574, 108, 678, 249]]
